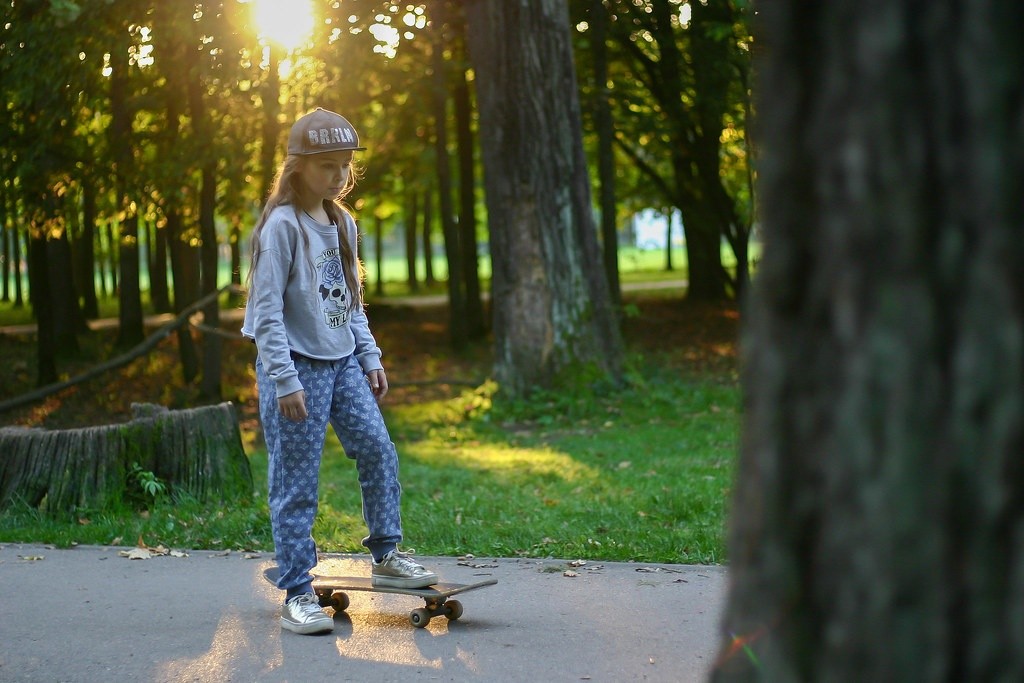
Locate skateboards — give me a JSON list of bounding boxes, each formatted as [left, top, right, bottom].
[[263, 566, 499, 628]]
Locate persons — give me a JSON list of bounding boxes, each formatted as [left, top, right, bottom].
[[240, 107, 438, 635]]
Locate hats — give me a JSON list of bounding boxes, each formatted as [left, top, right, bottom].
[[289, 107, 367, 155]]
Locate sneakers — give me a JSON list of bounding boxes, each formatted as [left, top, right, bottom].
[[371, 548, 438, 588], [281, 592, 334, 634]]
